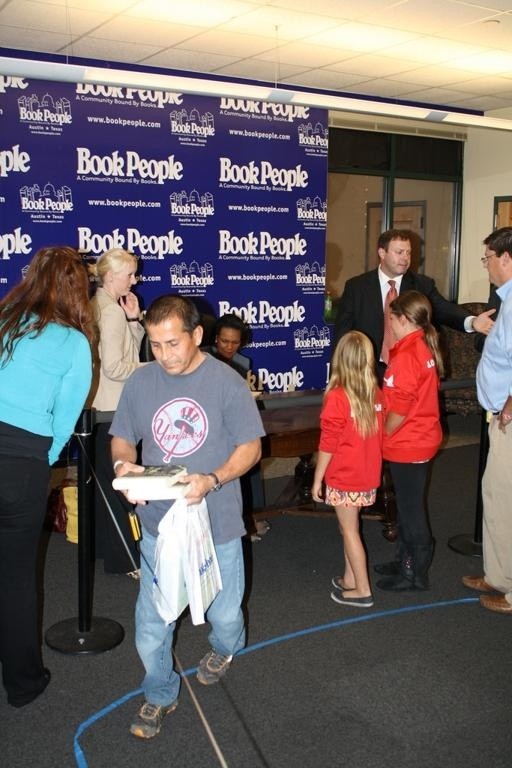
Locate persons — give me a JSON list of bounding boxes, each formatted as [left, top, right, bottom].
[[0, 246, 92, 708], [89, 249, 152, 573], [336, 230, 496, 365], [374, 290, 446, 594], [210, 314, 250, 379], [312, 331, 383, 606], [460, 227, 512, 613]]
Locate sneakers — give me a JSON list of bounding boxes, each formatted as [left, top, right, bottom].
[[129, 698, 179, 740], [196, 646, 233, 685]]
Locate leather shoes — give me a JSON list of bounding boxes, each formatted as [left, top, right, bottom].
[[375, 573, 425, 591], [8, 666, 51, 708], [461, 575, 495, 593], [479, 594, 512, 613], [374, 560, 401, 574]]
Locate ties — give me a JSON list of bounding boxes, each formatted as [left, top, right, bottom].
[[381, 280, 398, 365]]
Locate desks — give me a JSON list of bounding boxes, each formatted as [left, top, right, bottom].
[[254, 390, 326, 529]]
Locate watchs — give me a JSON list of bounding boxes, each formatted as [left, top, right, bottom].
[[209, 473, 221, 492]]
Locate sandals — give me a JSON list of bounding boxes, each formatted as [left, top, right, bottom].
[[332, 575, 350, 589], [330, 589, 374, 607]]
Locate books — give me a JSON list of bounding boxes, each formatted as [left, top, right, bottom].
[[111, 466, 187, 491], [128, 483, 194, 500]]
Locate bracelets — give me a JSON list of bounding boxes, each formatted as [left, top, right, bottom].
[[127, 318, 139, 321], [113, 459, 122, 472]]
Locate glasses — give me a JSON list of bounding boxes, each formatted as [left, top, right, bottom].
[[480, 254, 497, 263]]
